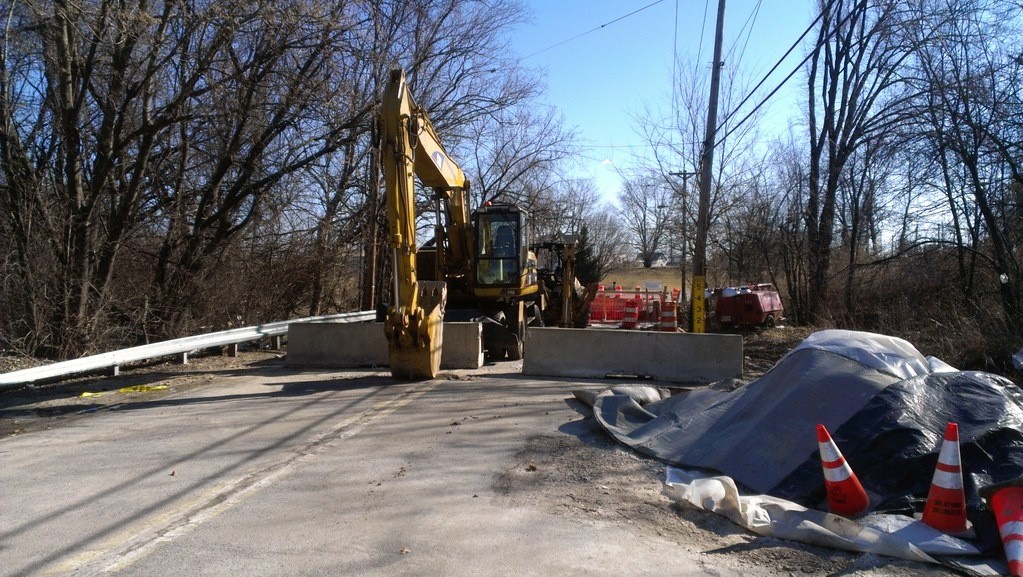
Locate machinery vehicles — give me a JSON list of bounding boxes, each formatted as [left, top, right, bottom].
[[379, 68, 546, 383]]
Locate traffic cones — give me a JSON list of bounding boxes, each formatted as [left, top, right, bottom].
[[914, 420, 981, 542], [815, 423, 873, 522], [978, 473, 1023, 577]]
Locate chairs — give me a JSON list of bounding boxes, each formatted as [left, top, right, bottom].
[[493, 225, 521, 256]]
